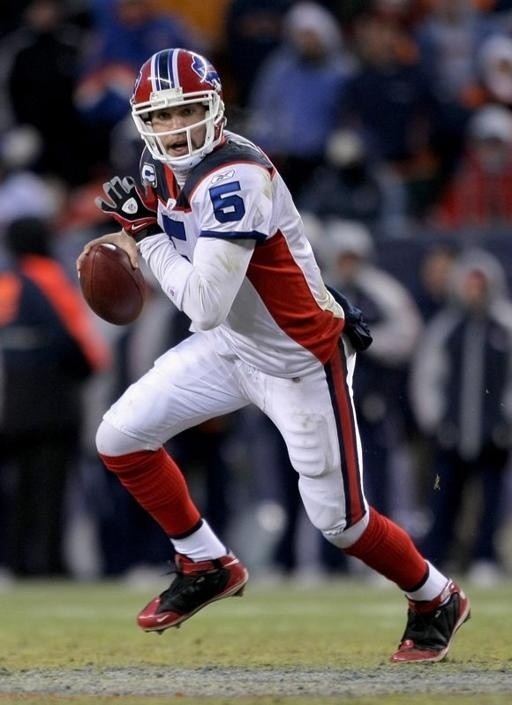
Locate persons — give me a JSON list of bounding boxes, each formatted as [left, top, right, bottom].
[[73, 45, 478, 666], [1, 0, 512, 594]]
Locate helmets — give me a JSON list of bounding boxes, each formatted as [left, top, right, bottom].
[[130, 48, 227, 175]]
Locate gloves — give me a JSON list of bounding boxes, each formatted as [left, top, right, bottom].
[[93, 174, 166, 242]]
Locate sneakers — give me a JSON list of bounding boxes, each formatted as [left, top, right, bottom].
[[390, 578, 472, 663], [137, 550, 251, 636]]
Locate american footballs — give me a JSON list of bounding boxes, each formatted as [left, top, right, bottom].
[[80, 242, 146, 325]]
